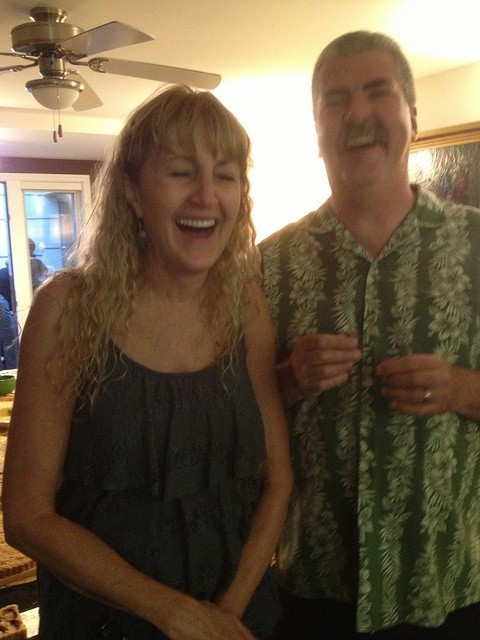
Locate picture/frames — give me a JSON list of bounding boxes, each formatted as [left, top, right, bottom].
[[410, 122, 479, 207]]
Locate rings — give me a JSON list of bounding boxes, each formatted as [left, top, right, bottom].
[[423, 386, 432, 400]]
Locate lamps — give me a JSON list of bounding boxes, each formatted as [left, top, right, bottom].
[[25, 78, 84, 110]]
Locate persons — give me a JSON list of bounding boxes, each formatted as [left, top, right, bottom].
[[2, 85, 293, 640], [0, 237, 45, 369], [255, 30, 480, 640]]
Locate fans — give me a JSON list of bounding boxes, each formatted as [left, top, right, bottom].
[[0, 8, 222, 142]]
